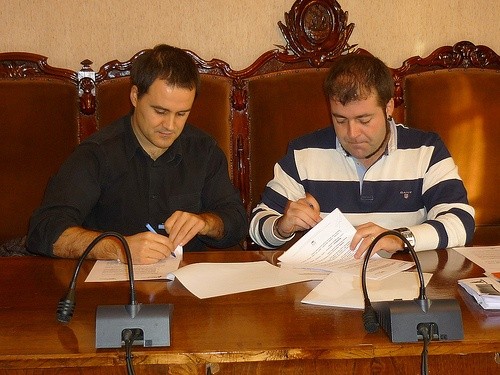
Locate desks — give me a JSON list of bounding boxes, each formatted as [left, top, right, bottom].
[[0, 250, 500, 375]]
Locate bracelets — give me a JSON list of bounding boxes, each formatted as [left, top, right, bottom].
[[393, 228, 416, 253]]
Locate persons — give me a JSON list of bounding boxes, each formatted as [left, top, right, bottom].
[[248, 55, 475, 260], [26, 43, 250, 265]]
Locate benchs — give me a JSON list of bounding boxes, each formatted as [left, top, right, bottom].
[[0, 0, 500, 244]]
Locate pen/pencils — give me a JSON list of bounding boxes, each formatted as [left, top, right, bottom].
[[145, 223, 177, 258]]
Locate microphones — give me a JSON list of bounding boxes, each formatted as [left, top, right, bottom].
[[361, 229, 465, 343], [55, 231, 172, 349]]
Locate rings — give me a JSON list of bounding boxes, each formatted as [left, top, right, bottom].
[[369, 235, 376, 241]]
